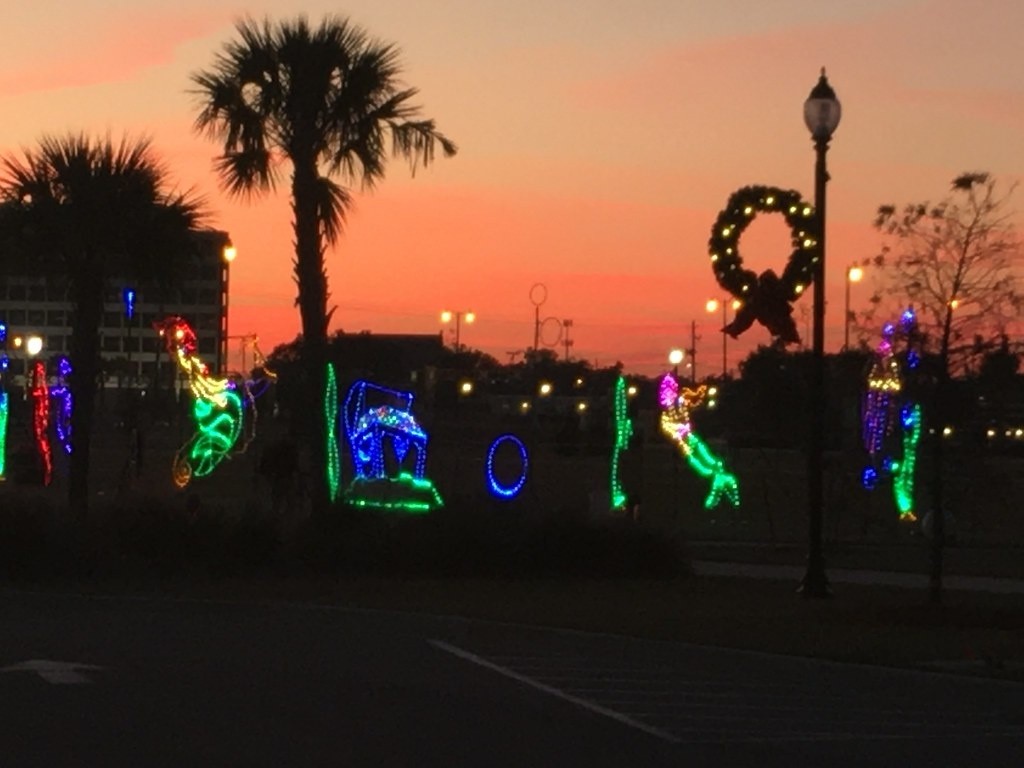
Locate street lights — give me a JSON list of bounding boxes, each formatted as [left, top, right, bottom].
[[220, 236, 236, 374], [671, 322, 702, 382], [12, 334, 38, 397], [843, 265, 867, 350], [439, 309, 476, 355], [802, 64, 841, 600], [706, 296, 742, 374]]
